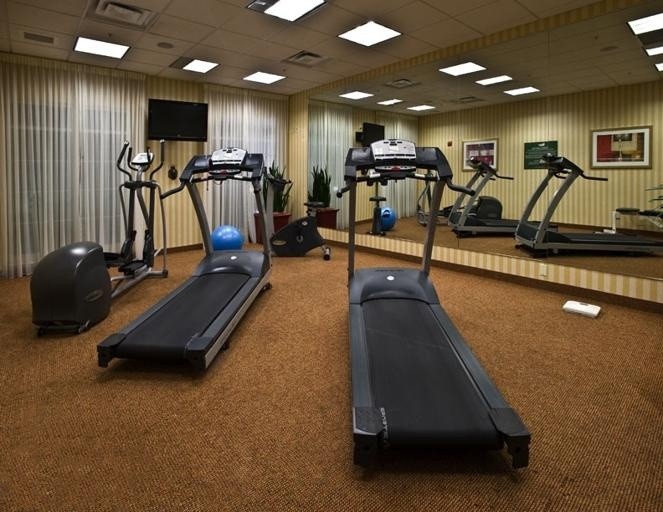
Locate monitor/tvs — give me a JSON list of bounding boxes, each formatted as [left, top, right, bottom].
[[361, 121, 385, 148], [146, 97, 208, 144]]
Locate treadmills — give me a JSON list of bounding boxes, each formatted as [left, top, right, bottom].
[[97, 147, 272, 373], [450, 158, 558, 239], [344, 138, 530, 468], [516, 153, 663, 259]]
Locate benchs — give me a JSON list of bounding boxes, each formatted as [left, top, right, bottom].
[[612, 206, 662, 234]]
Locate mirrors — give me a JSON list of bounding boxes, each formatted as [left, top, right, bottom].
[[303, 0, 662, 282]]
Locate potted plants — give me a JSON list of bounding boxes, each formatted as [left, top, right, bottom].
[[308, 165, 339, 229], [253, 159, 294, 245]]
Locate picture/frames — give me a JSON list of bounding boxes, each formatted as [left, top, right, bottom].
[[588, 125, 653, 170], [461, 137, 500, 172]]
